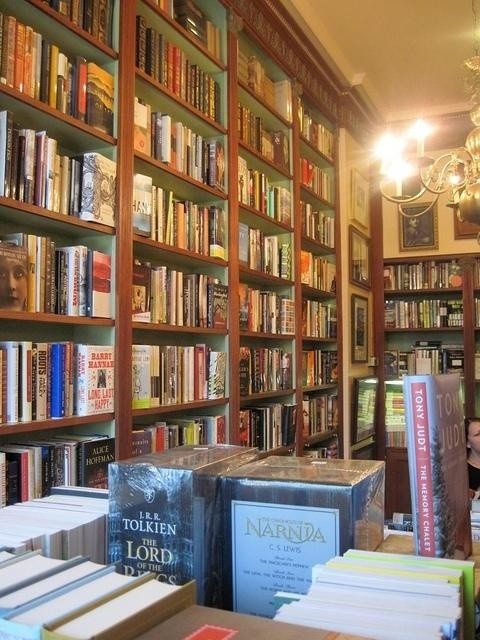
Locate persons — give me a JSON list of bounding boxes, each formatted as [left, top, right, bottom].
[[463, 418, 480, 492]]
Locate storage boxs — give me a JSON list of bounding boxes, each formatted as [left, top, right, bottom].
[[106, 444, 260, 613], [216, 457, 386, 618]]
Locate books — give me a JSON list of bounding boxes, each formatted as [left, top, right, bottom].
[[0, 2, 117, 424], [131, 3, 227, 455], [235, 34, 294, 446], [2, 435, 480, 638], [299, 98, 339, 460], [384, 262, 480, 377], [406, 375, 473, 558]]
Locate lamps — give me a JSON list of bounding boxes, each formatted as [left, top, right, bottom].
[[376, 0, 480, 247]]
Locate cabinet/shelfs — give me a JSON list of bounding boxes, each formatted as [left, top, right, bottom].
[[0, 0, 346, 518], [372, 251, 480, 523]]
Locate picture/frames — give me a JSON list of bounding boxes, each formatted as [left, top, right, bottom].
[[396, 201, 440, 252], [348, 223, 373, 292], [453, 206, 479, 241], [350, 293, 368, 363], [352, 376, 380, 445], [350, 167, 371, 230]]
